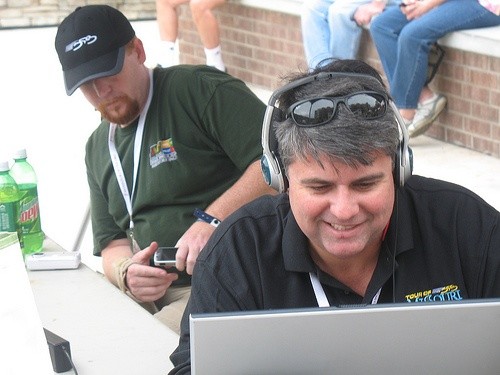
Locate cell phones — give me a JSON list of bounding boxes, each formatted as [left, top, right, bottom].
[[153, 246, 178, 267]]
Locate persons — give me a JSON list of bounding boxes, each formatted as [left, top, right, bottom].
[[153, 0, 247, 73], [300, 1, 500, 139], [54, 5, 278, 337], [167, 58, 500, 375]]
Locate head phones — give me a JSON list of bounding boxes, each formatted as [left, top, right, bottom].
[[260, 70, 414, 195]]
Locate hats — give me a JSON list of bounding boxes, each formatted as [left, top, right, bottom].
[[54, 4, 136, 95]]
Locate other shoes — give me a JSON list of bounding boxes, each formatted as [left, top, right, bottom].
[[406, 94, 446, 137]]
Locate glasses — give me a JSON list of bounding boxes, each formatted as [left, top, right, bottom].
[[284, 89, 390, 127]]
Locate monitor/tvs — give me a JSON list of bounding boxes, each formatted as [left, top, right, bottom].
[[188, 295, 500, 375]]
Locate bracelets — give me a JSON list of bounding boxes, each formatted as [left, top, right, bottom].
[[194, 208, 221, 227]]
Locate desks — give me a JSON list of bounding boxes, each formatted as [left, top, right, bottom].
[[20, 235, 181, 375]]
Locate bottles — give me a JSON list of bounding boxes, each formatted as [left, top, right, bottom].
[[9, 145, 44, 256], [0, 160, 24, 257]]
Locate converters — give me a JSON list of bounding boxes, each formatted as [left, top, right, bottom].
[[44, 328, 71, 373]]
